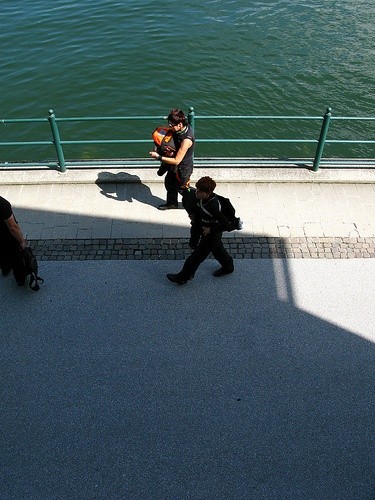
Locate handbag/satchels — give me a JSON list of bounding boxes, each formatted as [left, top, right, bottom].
[[20, 247, 38, 276]]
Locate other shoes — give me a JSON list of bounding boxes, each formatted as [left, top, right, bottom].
[[167, 273, 187, 285], [2, 268, 11, 276], [16, 279, 25, 286], [213, 265, 234, 277]]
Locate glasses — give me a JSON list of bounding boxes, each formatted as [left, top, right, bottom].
[[168, 121, 176, 128]]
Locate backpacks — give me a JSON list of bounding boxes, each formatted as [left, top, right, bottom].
[[214, 194, 240, 232]]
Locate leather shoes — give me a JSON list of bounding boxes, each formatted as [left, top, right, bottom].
[[157, 201, 178, 210]]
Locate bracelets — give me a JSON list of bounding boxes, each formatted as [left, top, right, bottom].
[[158, 155, 162, 161]]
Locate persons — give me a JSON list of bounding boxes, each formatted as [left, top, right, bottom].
[[0, 196, 26, 286], [149, 109, 195, 210], [166, 176, 235, 285]]
